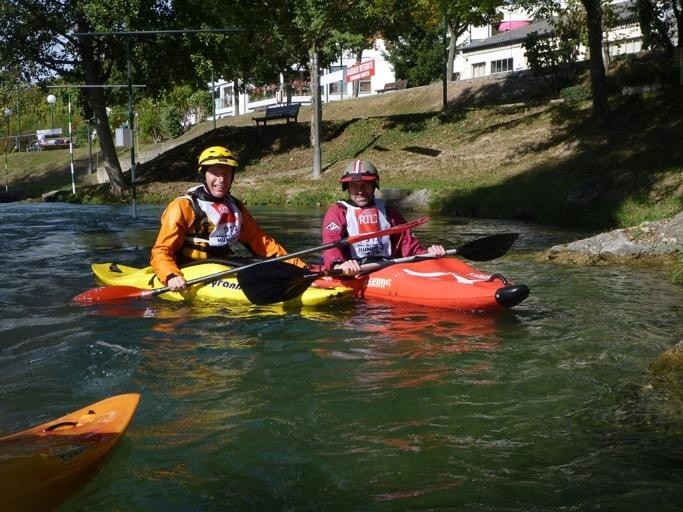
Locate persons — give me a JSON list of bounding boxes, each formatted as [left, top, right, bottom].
[[321, 160, 446, 279], [149, 146, 305, 292]]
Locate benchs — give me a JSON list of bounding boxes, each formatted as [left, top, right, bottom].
[[252, 103, 302, 126], [375, 80, 409, 94]]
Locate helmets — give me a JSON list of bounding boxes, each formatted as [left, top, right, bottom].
[[340, 161, 379, 191], [198, 146, 238, 174]]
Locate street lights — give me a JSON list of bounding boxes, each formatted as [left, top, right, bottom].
[[47, 94, 57, 129], [5, 107, 12, 136]]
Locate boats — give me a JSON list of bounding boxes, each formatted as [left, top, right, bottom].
[[308, 256, 531, 313], [0, 391, 143, 511], [90, 261, 355, 308]]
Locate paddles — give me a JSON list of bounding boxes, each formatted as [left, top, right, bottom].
[[236, 233, 518, 305], [72, 218, 432, 303]]
